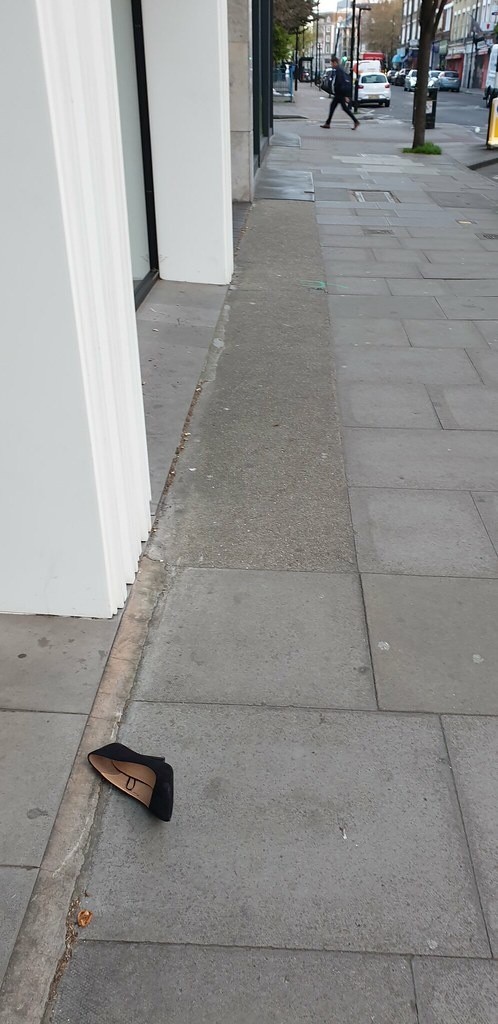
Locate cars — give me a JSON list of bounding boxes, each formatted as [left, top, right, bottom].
[[386, 68, 441, 91], [302, 50, 387, 96], [438, 70, 461, 92], [353, 71, 392, 108]]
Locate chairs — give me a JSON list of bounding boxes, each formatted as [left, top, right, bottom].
[[376, 77, 382, 81], [363, 78, 367, 83]]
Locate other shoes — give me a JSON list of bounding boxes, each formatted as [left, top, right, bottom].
[[320, 124, 330, 128], [352, 122, 360, 130]]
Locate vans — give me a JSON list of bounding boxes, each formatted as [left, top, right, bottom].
[[483, 43, 498, 107]]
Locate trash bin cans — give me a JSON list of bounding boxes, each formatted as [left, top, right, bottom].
[[411, 87, 438, 130]]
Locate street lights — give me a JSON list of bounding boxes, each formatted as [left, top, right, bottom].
[[352, 7, 372, 114]]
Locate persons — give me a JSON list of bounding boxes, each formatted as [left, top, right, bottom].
[[319, 56, 360, 131], [281, 63, 287, 81]]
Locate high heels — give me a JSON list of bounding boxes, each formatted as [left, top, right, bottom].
[[88, 741, 175, 821]]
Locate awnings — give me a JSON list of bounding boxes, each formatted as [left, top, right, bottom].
[[390, 55, 401, 62]]
